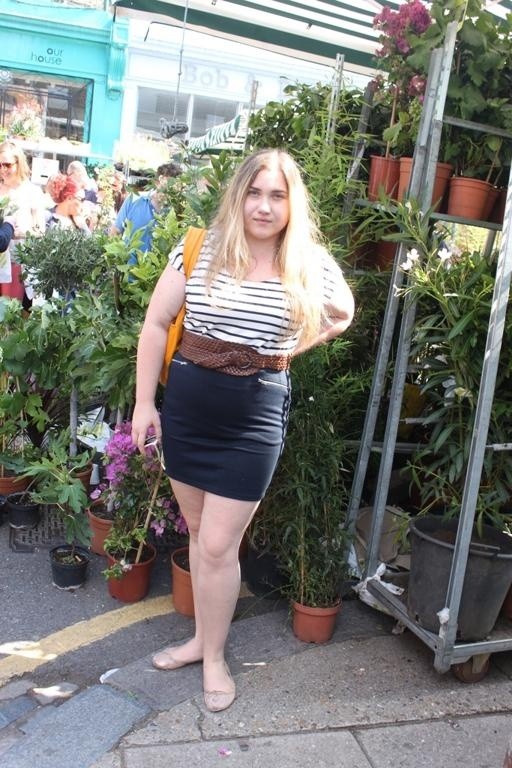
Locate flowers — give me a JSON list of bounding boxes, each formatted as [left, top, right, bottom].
[[362, 2, 429, 155], [104, 418, 188, 551], [362, 181, 511, 534]]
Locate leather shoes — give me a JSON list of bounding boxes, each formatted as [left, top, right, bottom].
[[202, 658, 235, 712], [152, 645, 206, 670]]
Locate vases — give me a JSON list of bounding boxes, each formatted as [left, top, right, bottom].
[[106, 543, 156, 603], [400, 511, 511, 641], [362, 154, 505, 217]]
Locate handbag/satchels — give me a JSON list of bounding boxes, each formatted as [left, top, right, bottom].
[[159, 318, 184, 388]]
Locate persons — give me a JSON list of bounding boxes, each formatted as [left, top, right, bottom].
[[2, 140, 184, 320], [131, 147, 357, 712]]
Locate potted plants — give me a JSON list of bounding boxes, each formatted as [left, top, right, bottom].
[[16, 430, 90, 591], [7, 415, 93, 542], [89, 428, 123, 557], [289, 337, 363, 641], [170, 546, 200, 615], [0, 337, 92, 497]]
